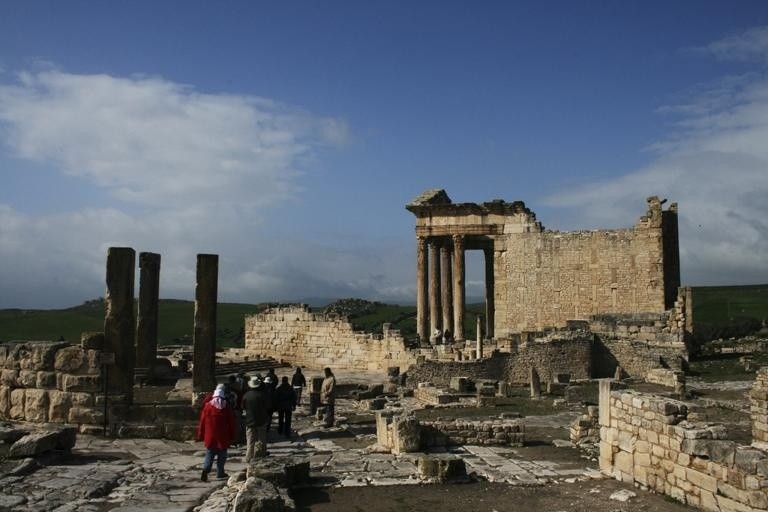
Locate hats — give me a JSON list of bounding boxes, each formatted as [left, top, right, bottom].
[[247, 376, 261, 389], [216, 383, 226, 391], [212, 388, 225, 398], [262, 376, 274, 385]]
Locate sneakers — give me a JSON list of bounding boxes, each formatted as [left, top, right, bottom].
[[200, 470, 208, 482], [217, 473, 228, 478]]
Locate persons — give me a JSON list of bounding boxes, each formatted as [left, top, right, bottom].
[[195, 368, 278, 482], [320, 368, 336, 427], [403, 332, 421, 349], [445, 328, 450, 344], [434, 327, 441, 345], [275, 376, 296, 436], [291, 366, 306, 405]]
